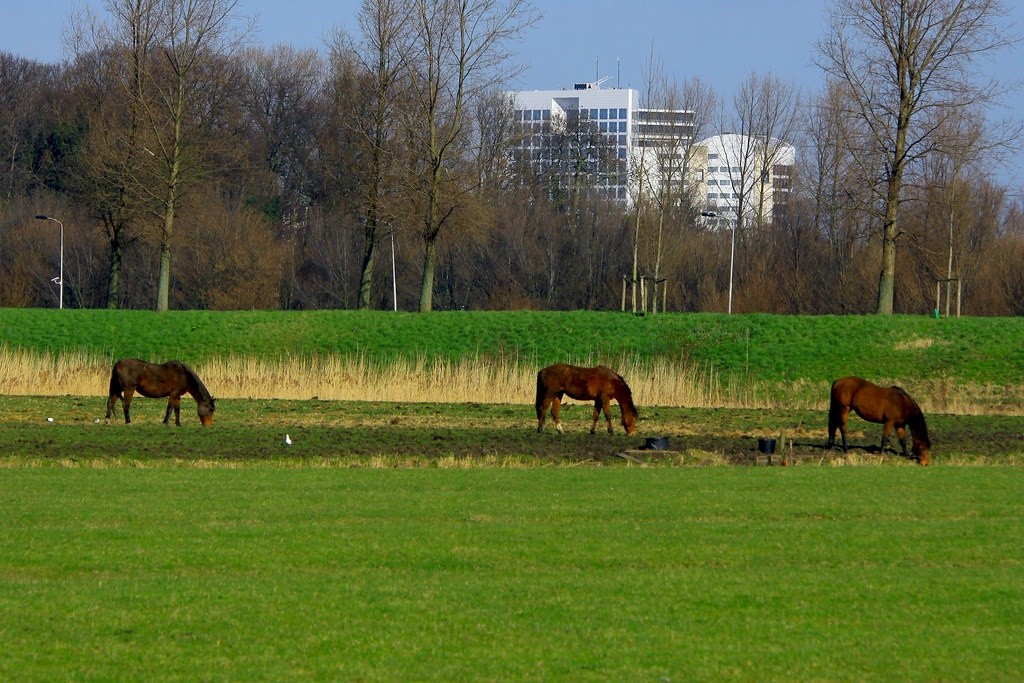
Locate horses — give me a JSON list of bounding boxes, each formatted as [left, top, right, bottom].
[[535, 364, 636, 437], [106, 358, 216, 427], [828, 377, 930, 466]]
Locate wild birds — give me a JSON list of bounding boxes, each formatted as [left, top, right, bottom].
[[285, 434, 292, 446]]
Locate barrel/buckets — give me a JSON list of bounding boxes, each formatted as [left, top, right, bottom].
[[645, 437, 668, 450], [757, 438, 777, 454]]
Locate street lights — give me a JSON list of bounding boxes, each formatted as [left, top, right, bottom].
[[358, 214, 398, 312], [699, 211, 735, 314], [34, 214, 65, 309]]
